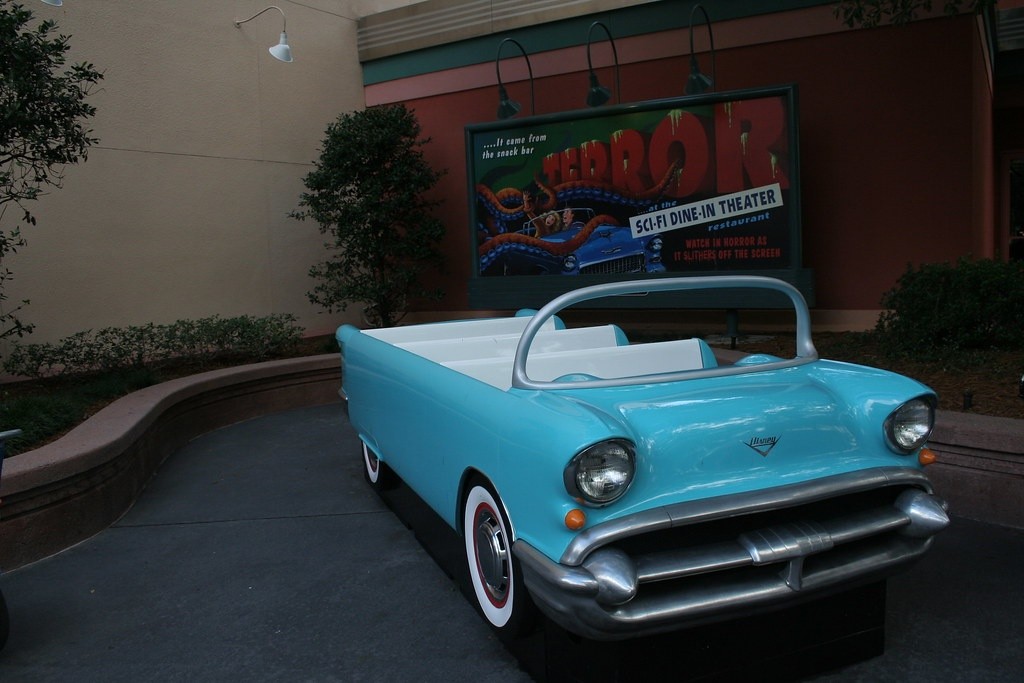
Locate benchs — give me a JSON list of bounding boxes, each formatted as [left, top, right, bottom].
[[399, 325, 631, 364], [358, 313, 564, 345], [443, 335, 717, 396]]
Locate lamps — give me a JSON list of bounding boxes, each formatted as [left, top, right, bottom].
[[685, 5, 716, 93], [234, 6, 294, 64], [496, 37, 534, 118], [586, 21, 620, 106]]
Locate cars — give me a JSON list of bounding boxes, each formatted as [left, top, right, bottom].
[[334, 274, 951, 647]]
